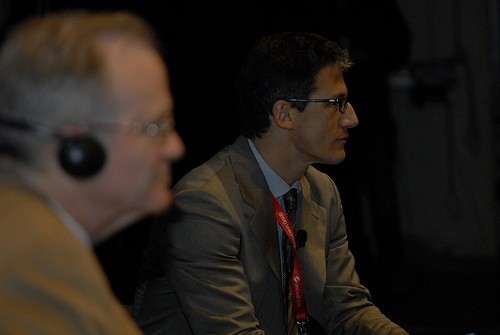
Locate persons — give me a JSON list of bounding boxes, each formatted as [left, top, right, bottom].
[[130, 31, 412, 335], [0, 13, 184, 335]]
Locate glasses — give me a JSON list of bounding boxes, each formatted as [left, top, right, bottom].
[[287, 94, 351, 112], [80, 113, 175, 144]]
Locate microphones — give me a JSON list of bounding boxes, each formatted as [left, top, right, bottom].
[[295, 229, 307, 247]]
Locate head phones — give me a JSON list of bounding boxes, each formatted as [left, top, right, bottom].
[[0, 118, 102, 178]]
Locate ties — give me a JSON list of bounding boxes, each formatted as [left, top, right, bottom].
[[282, 188, 299, 312]]
